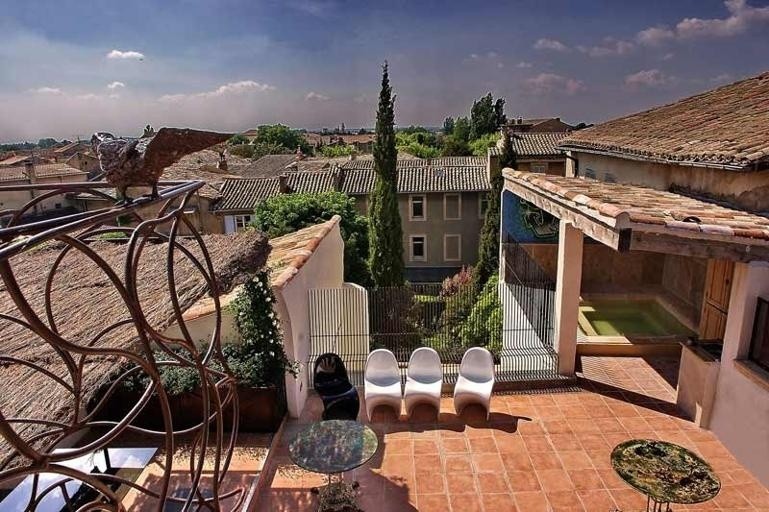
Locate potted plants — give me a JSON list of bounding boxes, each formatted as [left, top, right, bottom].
[[95, 273, 286, 435]]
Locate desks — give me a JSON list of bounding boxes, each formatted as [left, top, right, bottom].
[[287, 420, 379, 512]]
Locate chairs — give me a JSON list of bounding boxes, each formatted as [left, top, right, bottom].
[[305, 345, 496, 424]]
